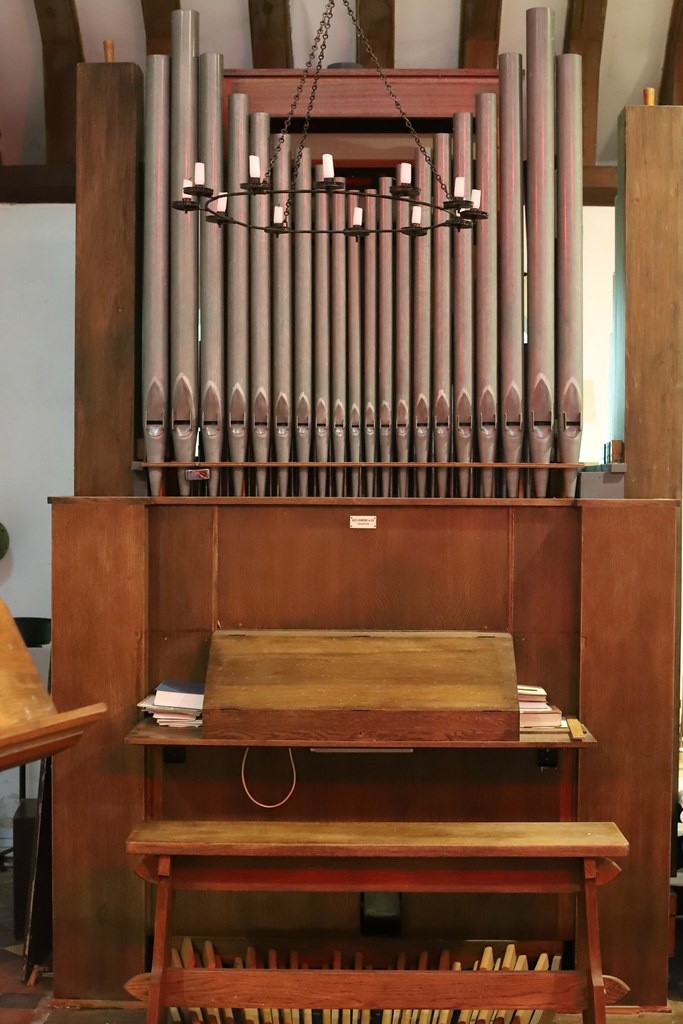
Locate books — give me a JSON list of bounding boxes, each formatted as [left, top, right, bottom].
[[165, 938, 565, 1024], [133, 678, 205, 730], [516, 682, 589, 736], [0, 794, 19, 867]]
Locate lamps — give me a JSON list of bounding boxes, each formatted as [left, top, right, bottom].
[[169, 1, 489, 235]]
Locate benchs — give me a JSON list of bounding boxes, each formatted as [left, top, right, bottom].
[[124, 820, 633, 1024]]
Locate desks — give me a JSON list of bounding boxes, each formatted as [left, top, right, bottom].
[[123, 710, 597, 972]]
